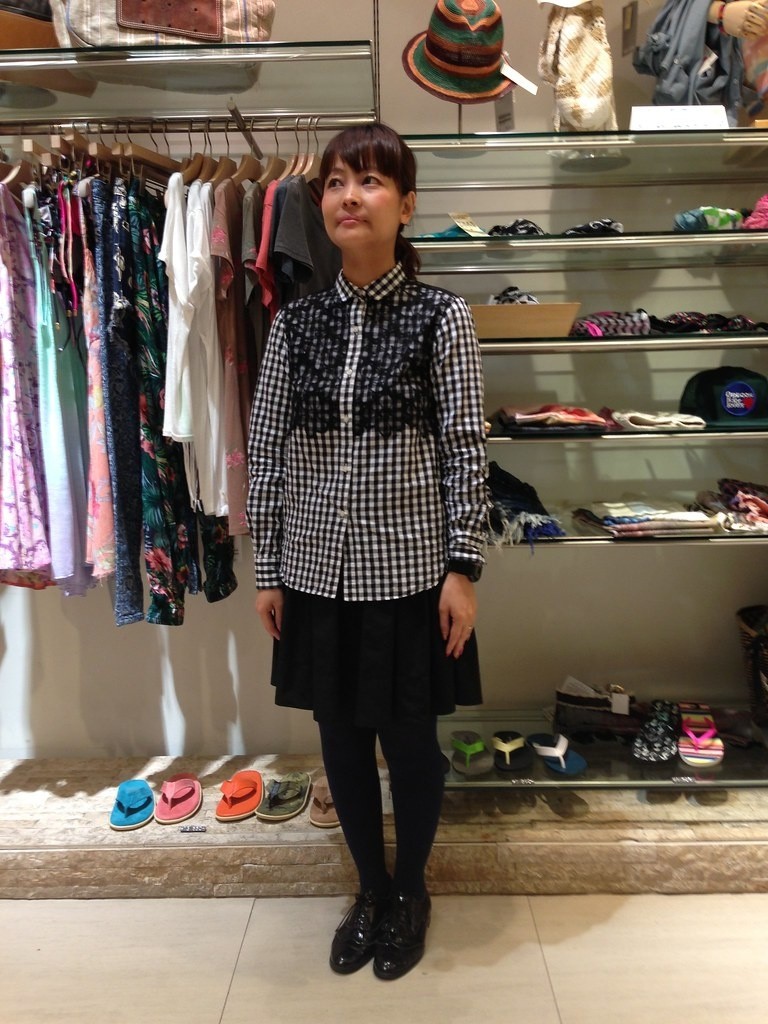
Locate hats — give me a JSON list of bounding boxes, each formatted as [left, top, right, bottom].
[[679, 364, 768, 433], [401, 0, 518, 106]]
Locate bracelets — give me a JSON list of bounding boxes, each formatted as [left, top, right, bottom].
[[717, 3, 734, 39]]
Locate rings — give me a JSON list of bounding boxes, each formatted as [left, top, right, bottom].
[[469, 627, 473, 629]]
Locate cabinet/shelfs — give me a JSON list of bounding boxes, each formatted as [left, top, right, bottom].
[[396, 129, 768, 792]]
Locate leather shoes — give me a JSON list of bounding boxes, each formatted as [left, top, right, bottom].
[[373, 888, 432, 980], [329, 887, 384, 975]]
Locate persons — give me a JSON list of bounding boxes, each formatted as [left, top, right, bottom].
[[632, 1, 768, 128], [245, 123, 486, 982]]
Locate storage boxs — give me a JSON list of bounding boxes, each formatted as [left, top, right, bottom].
[[469, 302, 582, 338]]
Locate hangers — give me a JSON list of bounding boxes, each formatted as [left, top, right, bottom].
[[0, 115, 324, 194]]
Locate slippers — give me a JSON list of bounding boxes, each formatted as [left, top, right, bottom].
[[255, 771, 311, 820], [309, 776, 341, 827], [154, 773, 204, 824], [527, 733, 588, 775], [452, 730, 494, 775], [632, 699, 682, 762], [215, 769, 263, 822], [554, 681, 637, 710], [110, 780, 156, 830], [490, 731, 533, 770], [677, 700, 724, 766]]
